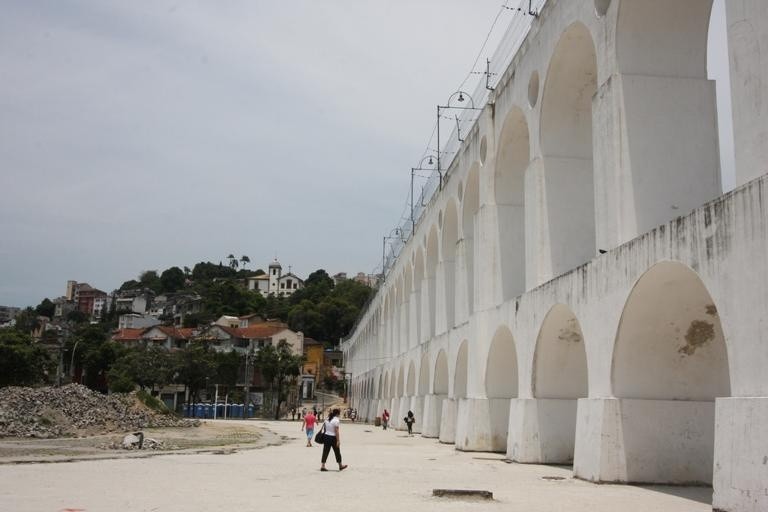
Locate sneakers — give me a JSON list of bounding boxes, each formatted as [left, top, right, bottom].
[[340, 465, 347, 470], [321, 468, 328, 470]]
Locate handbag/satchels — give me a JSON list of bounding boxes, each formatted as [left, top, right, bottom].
[[315, 432, 325, 444]]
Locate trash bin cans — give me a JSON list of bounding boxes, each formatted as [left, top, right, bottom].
[[376, 418, 380, 426], [182, 403, 254, 417]]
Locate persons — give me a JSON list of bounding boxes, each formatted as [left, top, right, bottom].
[[302, 411, 319, 447], [319, 409, 348, 471], [407, 410, 414, 435], [382, 408, 389, 430], [291, 407, 357, 423]]
